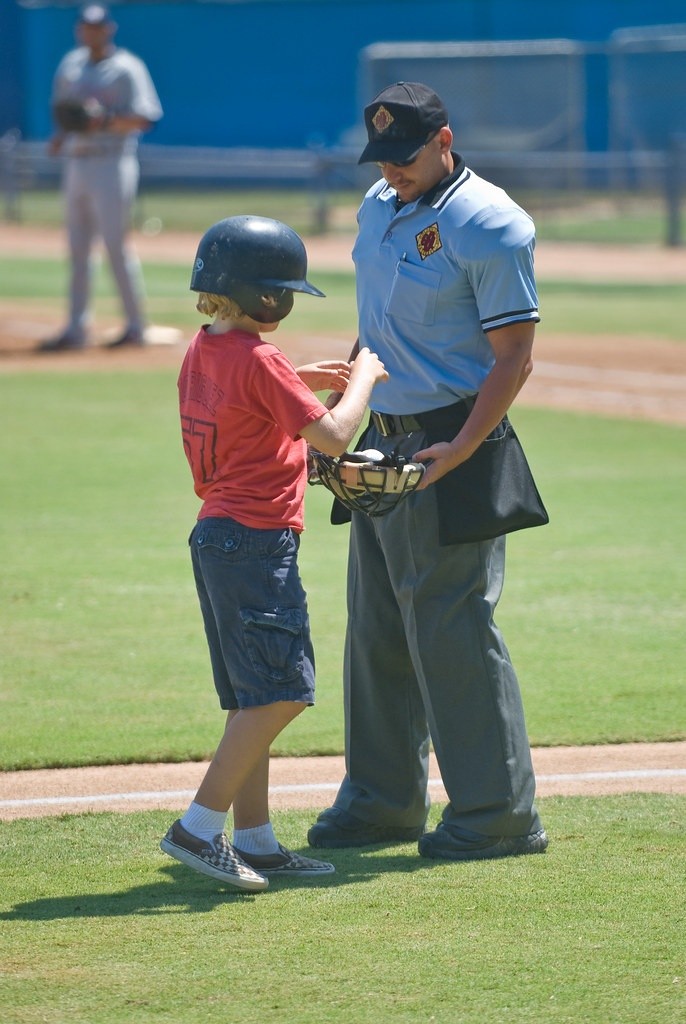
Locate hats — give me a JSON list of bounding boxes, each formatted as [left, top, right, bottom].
[[358, 81, 448, 166]]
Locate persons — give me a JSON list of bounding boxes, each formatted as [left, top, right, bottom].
[[40, 2, 163, 351], [160, 213, 390, 889], [307, 80, 547, 859]]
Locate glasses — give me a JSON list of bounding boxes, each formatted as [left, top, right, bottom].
[[376, 130, 437, 168]]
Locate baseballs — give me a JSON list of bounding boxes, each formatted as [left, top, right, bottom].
[[361, 448, 384, 462]]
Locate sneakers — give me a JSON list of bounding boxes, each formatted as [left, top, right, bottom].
[[307, 806, 425, 848], [162, 819, 269, 890], [417, 822, 549, 859], [232, 842, 336, 875]]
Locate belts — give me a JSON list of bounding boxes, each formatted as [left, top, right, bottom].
[[373, 395, 477, 438]]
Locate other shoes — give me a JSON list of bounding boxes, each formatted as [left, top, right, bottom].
[[41, 340, 97, 352], [108, 333, 146, 350]]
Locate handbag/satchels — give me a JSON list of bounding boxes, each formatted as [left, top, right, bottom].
[[429, 420, 550, 546]]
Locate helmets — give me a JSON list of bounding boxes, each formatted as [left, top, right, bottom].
[[189, 215, 326, 322]]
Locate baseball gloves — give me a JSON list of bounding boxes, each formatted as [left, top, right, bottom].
[[53, 94, 114, 135]]
